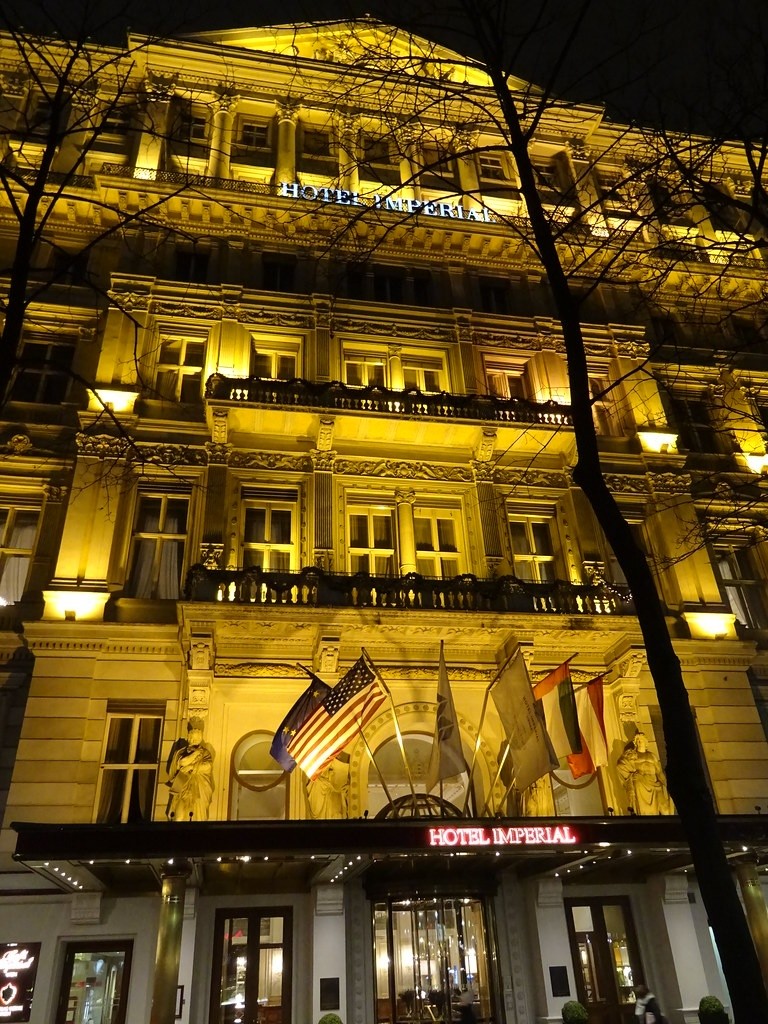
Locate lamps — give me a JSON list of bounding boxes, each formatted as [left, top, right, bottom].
[[754, 805, 761, 814], [170, 811, 175, 820], [715, 632, 727, 640], [608, 807, 614, 815], [189, 812, 193, 821], [627, 806, 637, 816], [363, 810, 369, 818], [64, 611, 76, 621]]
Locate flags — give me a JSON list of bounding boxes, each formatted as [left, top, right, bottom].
[[424, 650, 466, 795], [286, 656, 388, 782], [491, 649, 560, 793], [532, 661, 583, 758], [566, 677, 610, 780], [270, 671, 333, 773]]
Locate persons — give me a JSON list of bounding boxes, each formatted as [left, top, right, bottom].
[[615, 731, 675, 815], [168, 726, 214, 821], [635, 984, 665, 1024]]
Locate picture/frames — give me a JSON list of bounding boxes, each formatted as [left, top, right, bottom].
[[175, 985, 184, 1018]]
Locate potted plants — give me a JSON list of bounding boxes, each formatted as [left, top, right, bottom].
[[697, 996, 730, 1024]]
